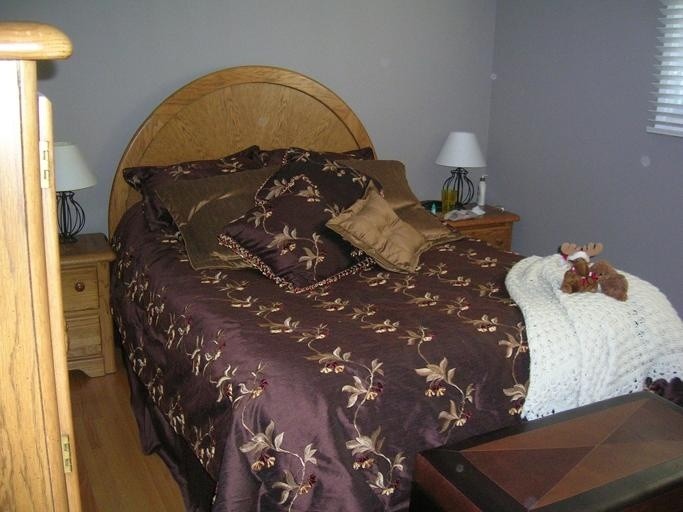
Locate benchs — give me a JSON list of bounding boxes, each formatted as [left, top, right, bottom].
[[408, 390, 683, 511]]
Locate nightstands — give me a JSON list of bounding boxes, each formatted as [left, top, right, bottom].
[[423, 203, 521, 252], [57, 233, 119, 381]]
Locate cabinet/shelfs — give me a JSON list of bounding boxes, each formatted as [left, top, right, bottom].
[[0, 20, 83, 512]]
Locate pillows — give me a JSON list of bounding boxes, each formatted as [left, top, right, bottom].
[[155, 166, 282, 271], [216, 175, 372, 294], [255, 145, 385, 205], [334, 158, 418, 211], [393, 202, 463, 245], [122, 144, 260, 237], [323, 147, 375, 165], [326, 180, 430, 275]]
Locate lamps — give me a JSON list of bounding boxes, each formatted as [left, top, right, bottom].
[[53, 141, 99, 245], [434, 131, 486, 212]]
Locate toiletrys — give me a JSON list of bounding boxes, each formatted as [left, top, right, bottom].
[[477, 174, 488, 206]]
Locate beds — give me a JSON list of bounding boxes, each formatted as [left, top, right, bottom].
[[109, 64, 669, 511]]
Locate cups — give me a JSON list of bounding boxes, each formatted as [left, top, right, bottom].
[[440, 189, 459, 216]]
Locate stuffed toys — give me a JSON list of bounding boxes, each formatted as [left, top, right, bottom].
[[643, 374, 682, 408], [558, 240, 605, 264], [560, 259, 628, 301]]
